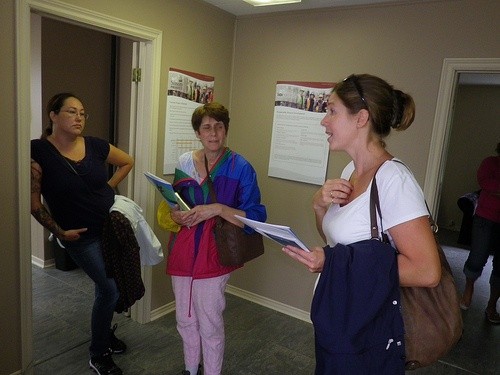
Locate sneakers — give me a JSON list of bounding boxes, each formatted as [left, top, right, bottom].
[[89, 353, 123, 375], [107, 322, 127, 353]]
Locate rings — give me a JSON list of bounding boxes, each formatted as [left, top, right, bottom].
[[329, 193, 334, 198]]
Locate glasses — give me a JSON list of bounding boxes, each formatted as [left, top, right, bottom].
[[60, 108, 90, 121], [342, 73, 369, 110]]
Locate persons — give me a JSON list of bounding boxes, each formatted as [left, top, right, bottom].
[[30, 91, 136, 375], [186, 80, 214, 102], [280, 74, 442, 375], [157, 103, 266, 375], [459, 143, 500, 323], [296, 89, 330, 113]]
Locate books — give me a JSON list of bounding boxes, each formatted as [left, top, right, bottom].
[[144, 171, 191, 212], [234, 214, 312, 253]]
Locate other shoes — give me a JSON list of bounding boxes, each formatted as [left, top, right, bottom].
[[484, 309, 500, 325], [177, 363, 202, 375], [459, 300, 472, 311]]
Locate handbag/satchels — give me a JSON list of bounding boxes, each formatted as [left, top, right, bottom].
[[370, 159, 465, 370], [214, 221, 265, 268]]
